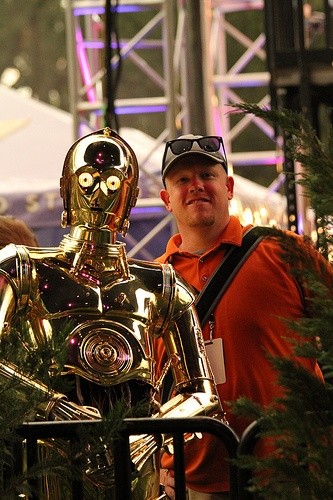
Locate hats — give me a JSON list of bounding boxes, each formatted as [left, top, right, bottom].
[[162, 133, 227, 190]]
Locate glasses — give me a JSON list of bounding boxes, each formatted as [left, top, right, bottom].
[[162, 136, 227, 174]]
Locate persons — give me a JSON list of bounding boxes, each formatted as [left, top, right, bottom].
[[137, 133, 333, 500], [1, 213, 55, 348]]
